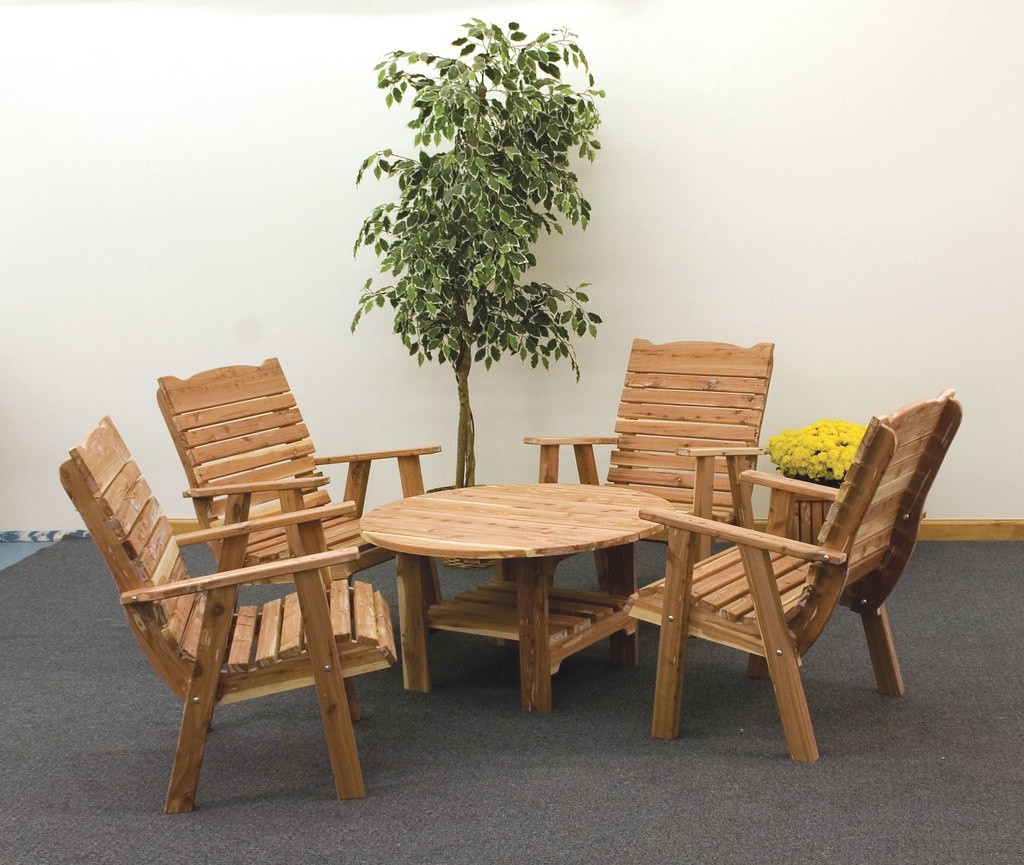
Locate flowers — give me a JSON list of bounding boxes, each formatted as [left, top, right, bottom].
[[764, 418, 867, 483]]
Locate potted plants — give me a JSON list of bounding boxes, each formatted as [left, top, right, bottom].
[[352, 17, 605, 573]]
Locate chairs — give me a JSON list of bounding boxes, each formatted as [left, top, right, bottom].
[[529, 338, 776, 587], [623, 388, 963, 765], [157, 357, 444, 636], [58, 415, 397, 815]]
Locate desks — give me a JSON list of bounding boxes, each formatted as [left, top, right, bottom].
[[360, 483, 674, 714]]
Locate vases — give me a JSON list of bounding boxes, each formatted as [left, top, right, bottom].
[[783, 471, 844, 488]]
